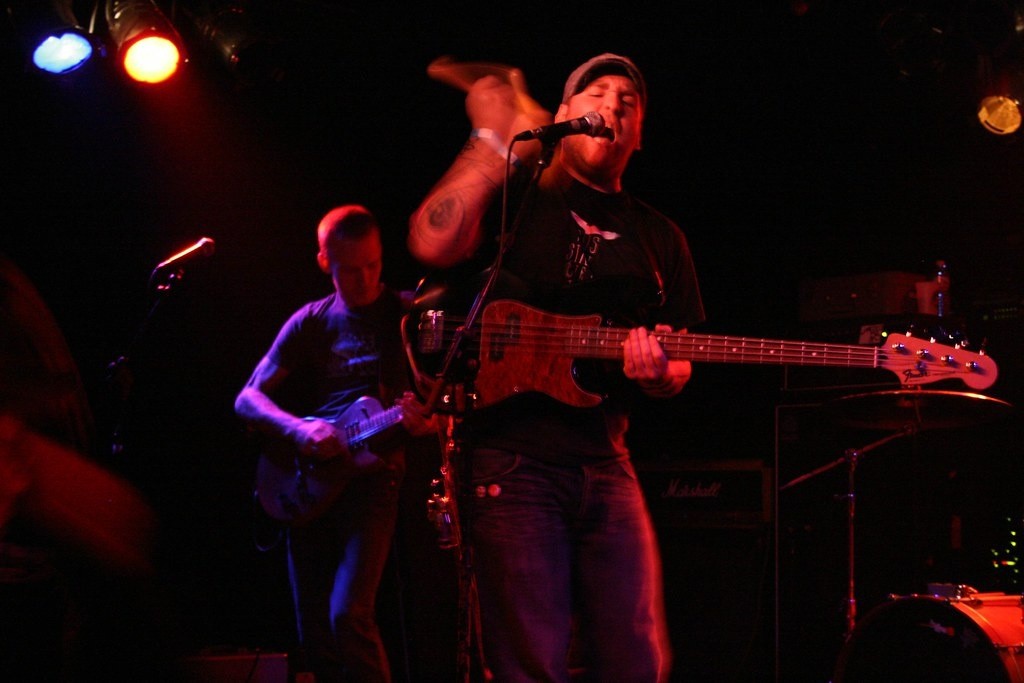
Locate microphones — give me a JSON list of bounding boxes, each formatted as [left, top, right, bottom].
[[514, 112, 606, 141], [155, 238, 215, 273]]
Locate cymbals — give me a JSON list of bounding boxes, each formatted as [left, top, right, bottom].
[[815, 386, 1014, 429]]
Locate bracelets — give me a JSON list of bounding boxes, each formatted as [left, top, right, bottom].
[[472, 124, 514, 170]]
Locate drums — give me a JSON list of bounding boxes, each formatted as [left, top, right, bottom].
[[833, 582, 1024, 683]]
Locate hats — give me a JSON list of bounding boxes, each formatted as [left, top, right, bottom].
[[562, 53, 647, 113]]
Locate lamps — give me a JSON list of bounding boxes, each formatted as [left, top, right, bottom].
[[977, 58, 1024, 136], [181, 0, 275, 75], [7, 0, 100, 78], [105, 0, 190, 91]]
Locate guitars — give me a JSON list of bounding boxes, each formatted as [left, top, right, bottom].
[[406, 267, 999, 417], [255, 393, 405, 527]]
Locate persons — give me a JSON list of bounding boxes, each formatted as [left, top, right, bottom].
[[2, 198, 444, 683], [411, 56, 706, 683]]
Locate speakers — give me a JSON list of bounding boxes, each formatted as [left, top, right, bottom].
[[638, 403, 996, 683]]
